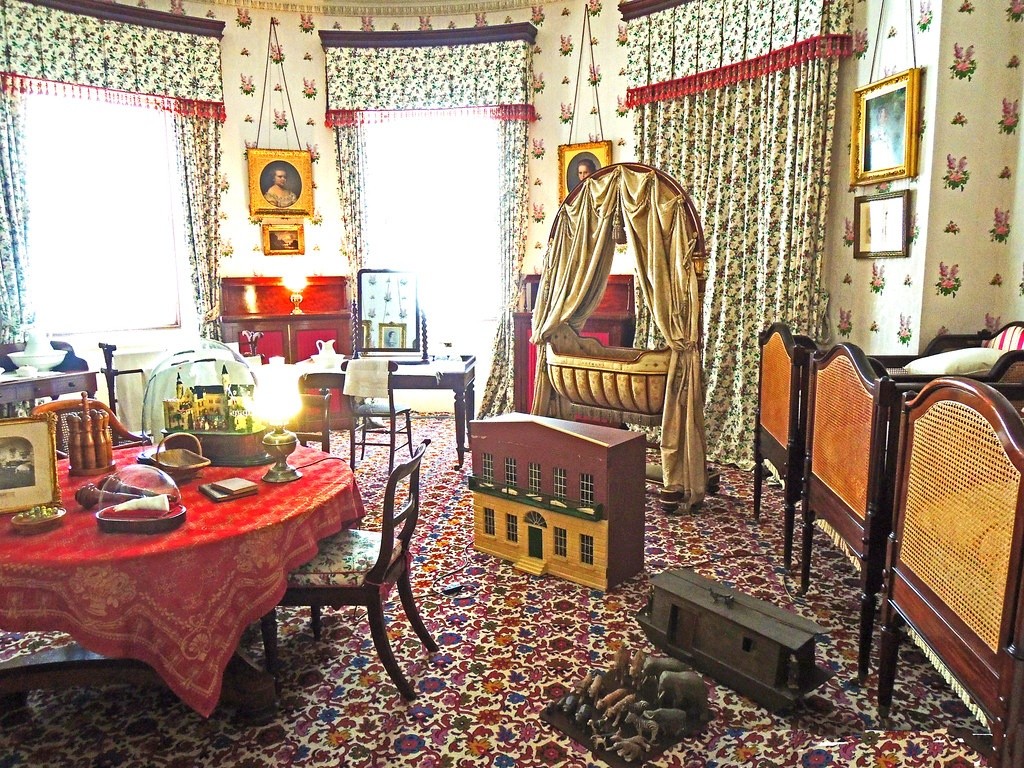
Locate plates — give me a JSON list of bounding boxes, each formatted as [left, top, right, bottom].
[[11, 508, 67, 533]]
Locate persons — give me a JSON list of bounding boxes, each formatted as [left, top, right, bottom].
[[2, 446, 34, 472], [264, 165, 298, 207], [569, 158, 597, 193], [868, 107, 896, 169]]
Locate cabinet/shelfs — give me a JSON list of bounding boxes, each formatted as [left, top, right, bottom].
[[218, 274, 356, 433], [512, 275, 636, 424]]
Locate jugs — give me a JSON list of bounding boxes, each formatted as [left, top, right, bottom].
[[316, 340, 336, 369], [18, 322, 55, 350]]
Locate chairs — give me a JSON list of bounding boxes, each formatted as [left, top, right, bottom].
[[29, 399, 153, 458], [290, 393, 334, 453], [254, 438, 437, 702], [340, 358, 415, 474]]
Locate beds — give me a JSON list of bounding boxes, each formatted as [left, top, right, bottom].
[[753, 321, 1024, 768]]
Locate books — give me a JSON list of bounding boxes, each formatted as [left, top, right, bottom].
[[197, 483, 258, 502], [211, 476, 258, 496]]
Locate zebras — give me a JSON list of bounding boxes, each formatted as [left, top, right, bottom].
[[624, 711, 659, 742]]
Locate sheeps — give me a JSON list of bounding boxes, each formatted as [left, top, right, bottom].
[[611, 644, 629, 685], [544, 695, 591, 734], [629, 650, 646, 686]]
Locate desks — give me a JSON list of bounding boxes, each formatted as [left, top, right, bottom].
[[0, 437, 365, 729], [292, 354, 476, 470], [0, 370, 99, 453]]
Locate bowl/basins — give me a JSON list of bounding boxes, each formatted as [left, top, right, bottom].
[[310, 354, 345, 368], [7, 351, 68, 373], [16, 365, 38, 376]]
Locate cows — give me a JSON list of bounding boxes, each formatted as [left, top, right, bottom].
[[640, 708, 687, 737]]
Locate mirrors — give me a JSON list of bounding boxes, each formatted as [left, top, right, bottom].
[[352, 269, 432, 364]]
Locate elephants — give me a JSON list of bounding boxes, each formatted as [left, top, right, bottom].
[[655, 669, 708, 721], [640, 656, 684, 686]]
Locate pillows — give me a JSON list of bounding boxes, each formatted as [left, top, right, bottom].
[[903, 347, 1009, 377]]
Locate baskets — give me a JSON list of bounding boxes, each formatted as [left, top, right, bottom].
[[149, 432, 211, 482]]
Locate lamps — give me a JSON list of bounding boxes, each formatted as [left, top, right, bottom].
[[249, 356, 304, 484], [287, 270, 308, 316]]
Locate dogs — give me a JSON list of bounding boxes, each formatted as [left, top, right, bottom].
[[586, 717, 608, 734], [591, 734, 608, 751]]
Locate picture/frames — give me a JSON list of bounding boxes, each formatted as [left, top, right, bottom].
[[247, 147, 314, 219], [557, 140, 612, 209], [851, 67, 921, 187], [262, 223, 306, 254], [0, 411, 63, 516], [853, 189, 911, 259], [378, 322, 406, 348]]
